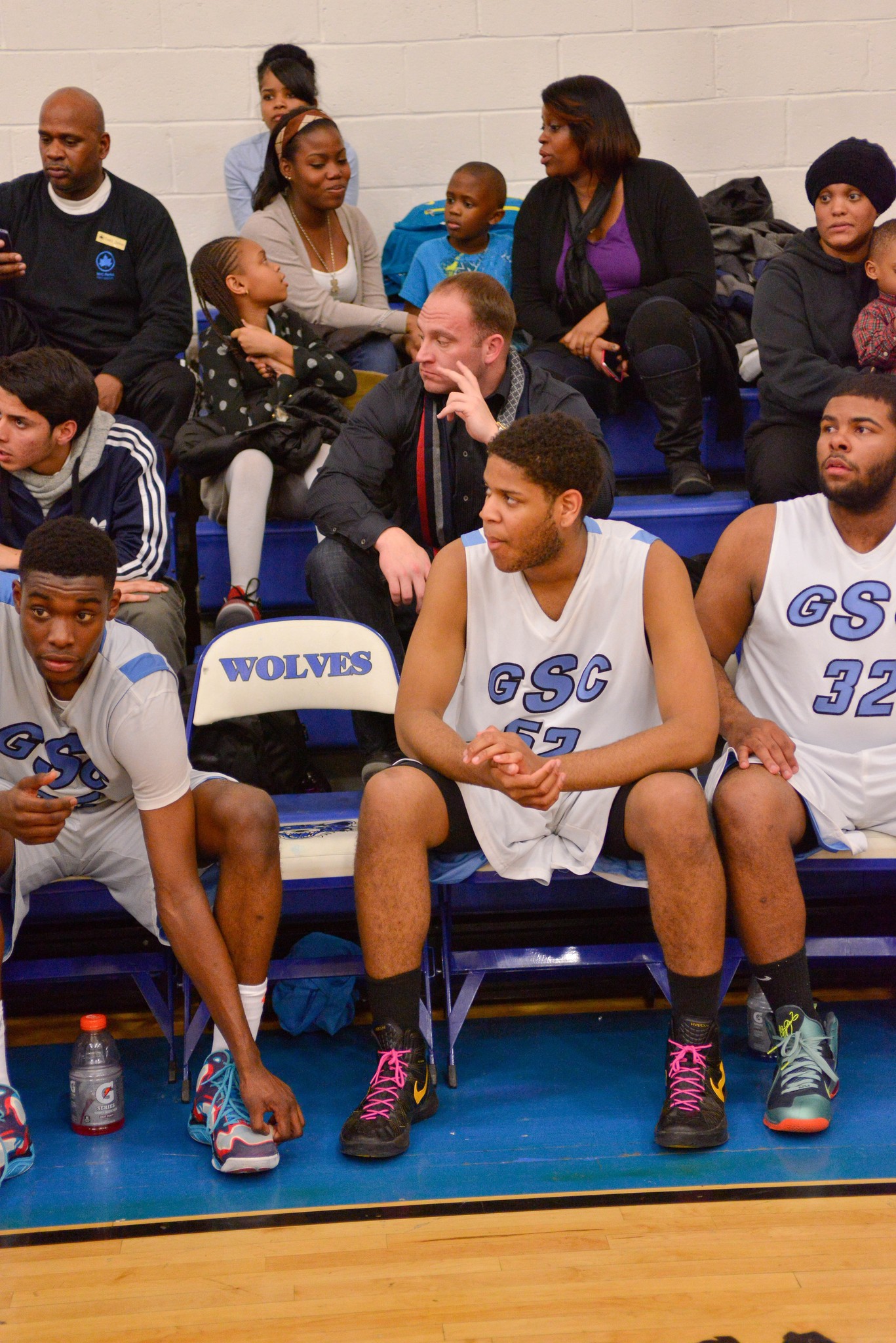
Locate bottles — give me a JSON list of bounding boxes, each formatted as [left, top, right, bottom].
[[69, 1014, 124, 1135]]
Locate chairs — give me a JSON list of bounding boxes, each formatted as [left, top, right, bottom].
[[0, 615, 896, 1100]]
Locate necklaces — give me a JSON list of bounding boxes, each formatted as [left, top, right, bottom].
[[286, 193, 342, 296]]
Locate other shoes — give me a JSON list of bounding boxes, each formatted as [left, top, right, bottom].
[[360, 750, 398, 784]]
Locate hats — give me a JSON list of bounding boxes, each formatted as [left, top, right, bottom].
[[805, 137, 896, 215]]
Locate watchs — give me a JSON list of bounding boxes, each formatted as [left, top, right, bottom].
[[496, 421, 507, 433]]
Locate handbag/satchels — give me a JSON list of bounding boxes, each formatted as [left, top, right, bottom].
[[376, 196, 526, 294]]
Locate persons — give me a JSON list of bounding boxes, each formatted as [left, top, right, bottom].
[[743, 136, 896, 505], [191, 236, 358, 636], [0, 86, 197, 454], [338, 412, 727, 1157], [308, 271, 615, 786], [224, 44, 743, 495], [0, 515, 307, 1185], [0, 347, 187, 673], [693, 372, 896, 1133]]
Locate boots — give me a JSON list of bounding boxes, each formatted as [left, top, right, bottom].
[[641, 359, 716, 496]]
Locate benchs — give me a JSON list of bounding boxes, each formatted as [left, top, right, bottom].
[[167, 311, 764, 617]]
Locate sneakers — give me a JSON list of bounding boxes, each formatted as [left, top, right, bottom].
[[213, 586, 262, 636], [0, 1083, 36, 1182], [337, 1019, 438, 1158], [655, 1009, 728, 1149], [185, 1050, 280, 1175], [761, 1003, 840, 1132]]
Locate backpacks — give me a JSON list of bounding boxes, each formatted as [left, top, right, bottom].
[[175, 660, 338, 795]]
